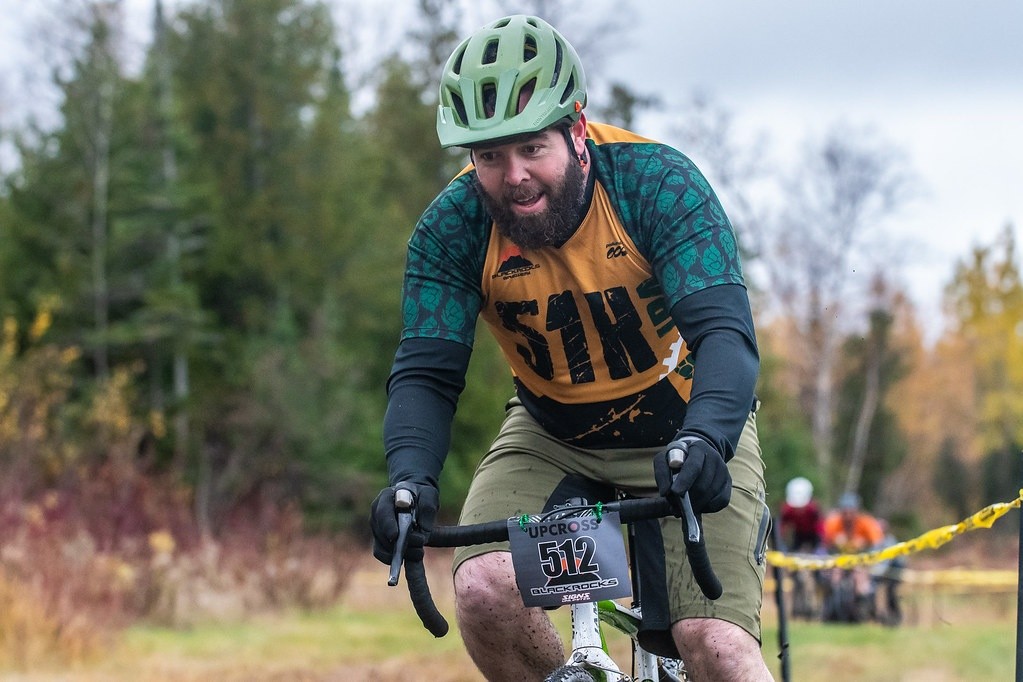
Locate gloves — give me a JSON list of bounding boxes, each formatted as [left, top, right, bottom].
[[368, 479, 440, 565], [652, 435, 732, 515]]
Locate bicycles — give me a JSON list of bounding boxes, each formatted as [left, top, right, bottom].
[[388, 439, 723, 682], [774, 536, 907, 625]]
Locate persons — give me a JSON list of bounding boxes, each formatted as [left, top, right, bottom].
[[370, 15, 777, 682], [770, 476, 905, 624]]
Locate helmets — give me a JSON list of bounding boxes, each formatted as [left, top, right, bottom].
[[435, 14, 588, 150], [836, 493, 862, 510], [785, 477, 814, 508]]
